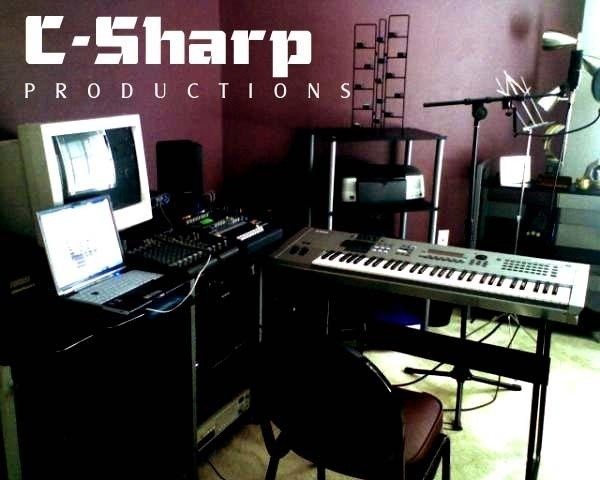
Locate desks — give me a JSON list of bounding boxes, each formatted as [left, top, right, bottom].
[[12, 196, 308, 480]]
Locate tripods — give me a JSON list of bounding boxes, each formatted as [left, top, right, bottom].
[[405, 90, 571, 431]]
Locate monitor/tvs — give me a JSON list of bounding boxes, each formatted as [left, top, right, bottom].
[[0, 113, 154, 255]]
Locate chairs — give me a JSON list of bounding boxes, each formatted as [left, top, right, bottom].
[[259, 334, 450, 480]]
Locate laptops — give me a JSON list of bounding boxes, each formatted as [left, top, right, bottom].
[[34, 194, 185, 316]]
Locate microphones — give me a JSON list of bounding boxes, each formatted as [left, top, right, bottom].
[[538, 82, 573, 111]]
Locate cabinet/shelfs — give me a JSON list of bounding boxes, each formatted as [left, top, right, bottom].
[[292, 125, 447, 330], [476, 157, 599, 342]]
